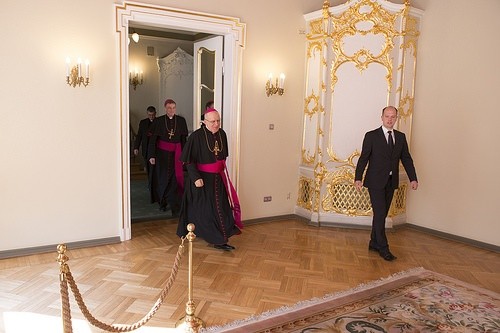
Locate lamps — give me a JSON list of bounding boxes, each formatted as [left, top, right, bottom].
[[130, 71, 144, 90], [265, 73, 285, 98], [66, 61, 90, 88]]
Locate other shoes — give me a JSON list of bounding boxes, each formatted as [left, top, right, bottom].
[[214, 244, 235, 251], [160, 207, 166, 211], [368, 246, 397, 261]]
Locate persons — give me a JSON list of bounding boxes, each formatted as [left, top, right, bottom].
[[148, 99, 188, 218], [201, 100, 214, 126], [133, 105, 158, 191], [353, 106, 418, 262], [175, 108, 244, 250]]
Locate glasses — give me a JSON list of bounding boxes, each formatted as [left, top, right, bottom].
[[204, 119, 221, 123]]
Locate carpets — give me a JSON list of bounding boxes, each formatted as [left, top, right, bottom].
[[191, 266, 500, 333]]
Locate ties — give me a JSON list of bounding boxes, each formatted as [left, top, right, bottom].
[[387, 131, 394, 153]]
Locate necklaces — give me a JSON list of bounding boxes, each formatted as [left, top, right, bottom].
[[203, 126, 223, 155], [165, 113, 176, 139]]
